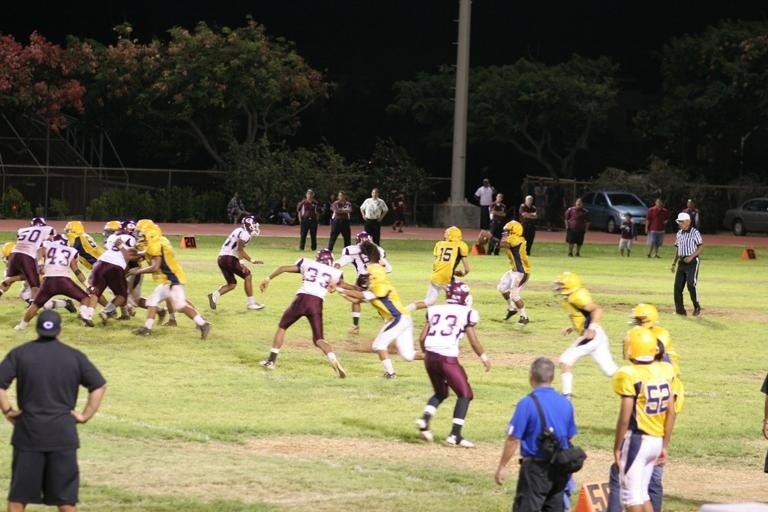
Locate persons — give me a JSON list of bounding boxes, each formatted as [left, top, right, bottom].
[[495, 357, 577, 512], [759, 372, 768, 473], [210, 217, 680, 379], [613, 326, 676, 511], [226, 188, 405, 252], [674, 212, 701, 318], [475, 179, 698, 258], [414, 281, 492, 448], [1, 216, 213, 341], [606, 337, 684, 511], [0, 309, 107, 512]]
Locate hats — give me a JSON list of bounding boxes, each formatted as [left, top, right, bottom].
[[37, 310, 60, 337], [676, 212, 690, 224]]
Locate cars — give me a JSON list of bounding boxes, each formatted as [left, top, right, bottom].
[[723, 197, 767, 237], [579, 190, 650, 233]]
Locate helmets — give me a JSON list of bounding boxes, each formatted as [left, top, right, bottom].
[[554, 272, 581, 294], [628, 303, 658, 327], [446, 281, 473, 306], [444, 226, 462, 241], [139, 226, 161, 240], [623, 328, 657, 361], [368, 264, 386, 284], [104, 221, 121, 231], [64, 221, 84, 234], [316, 249, 334, 266], [504, 220, 524, 236], [136, 219, 153, 231], [357, 231, 372, 242], [2, 243, 15, 256], [120, 220, 135, 231], [52, 234, 70, 246], [242, 216, 261, 235], [30, 218, 45, 227]]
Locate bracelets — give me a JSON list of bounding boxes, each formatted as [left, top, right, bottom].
[[3, 406, 12, 414]]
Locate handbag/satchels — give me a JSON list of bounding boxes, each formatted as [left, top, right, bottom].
[[537, 427, 587, 474]]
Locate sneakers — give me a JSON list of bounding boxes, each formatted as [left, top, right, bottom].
[[80, 312, 95, 326], [518, 315, 529, 325], [208, 293, 217, 309], [133, 327, 151, 336], [99, 310, 108, 325], [117, 314, 130, 320], [200, 322, 210, 339], [447, 433, 474, 447], [259, 360, 275, 369], [332, 360, 345, 378], [382, 372, 396, 380], [160, 320, 176, 327], [248, 302, 265, 309], [693, 307, 699, 316], [503, 309, 517, 320], [415, 418, 432, 440], [157, 307, 166, 323], [64, 300, 76, 312], [674, 310, 686, 315]]
